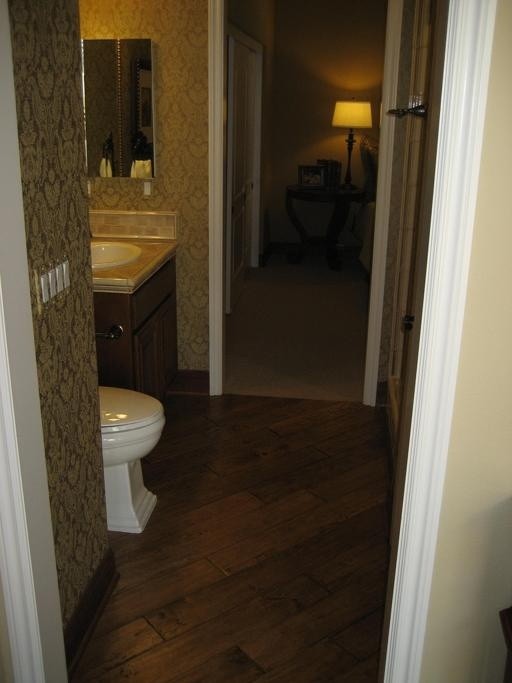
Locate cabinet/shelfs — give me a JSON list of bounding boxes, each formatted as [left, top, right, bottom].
[[94, 258, 178, 403]]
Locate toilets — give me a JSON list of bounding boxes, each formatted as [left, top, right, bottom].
[[98, 386, 167, 532]]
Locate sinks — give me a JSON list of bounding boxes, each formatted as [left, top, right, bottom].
[[90, 239, 141, 269]]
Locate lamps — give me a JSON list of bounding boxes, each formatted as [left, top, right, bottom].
[[332, 100, 373, 190]]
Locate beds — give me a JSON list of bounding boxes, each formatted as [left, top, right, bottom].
[[346, 189, 379, 274]]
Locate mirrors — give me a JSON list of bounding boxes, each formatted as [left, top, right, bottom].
[[82, 40, 154, 181]]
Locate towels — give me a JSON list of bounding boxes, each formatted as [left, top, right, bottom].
[[99, 158, 113, 178], [130, 159, 152, 179]]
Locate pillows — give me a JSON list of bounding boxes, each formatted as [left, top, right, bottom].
[[359, 135, 379, 209]]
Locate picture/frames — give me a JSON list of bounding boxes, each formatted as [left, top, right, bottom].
[[298, 165, 329, 191], [317, 159, 341, 190], [131, 58, 152, 154]]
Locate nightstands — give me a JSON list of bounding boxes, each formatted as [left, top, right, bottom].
[[285, 184, 364, 271]]
